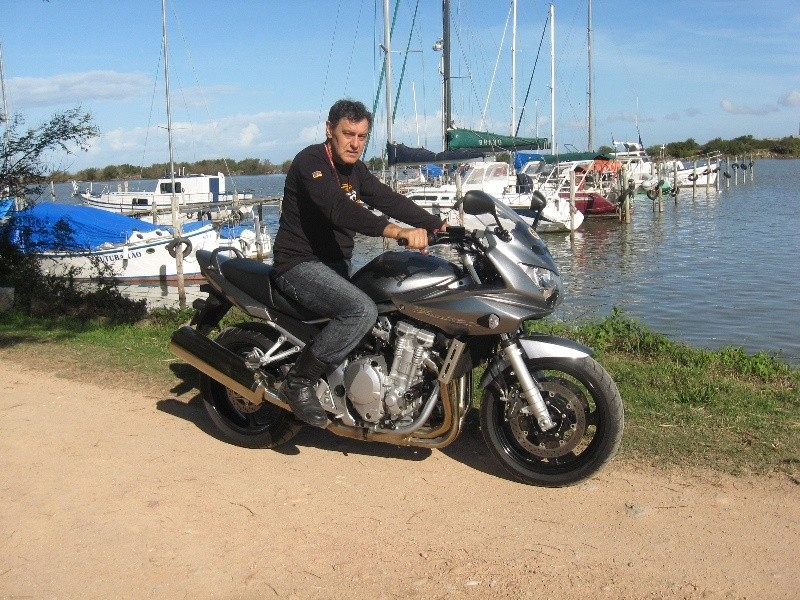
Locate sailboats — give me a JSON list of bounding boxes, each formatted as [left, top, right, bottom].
[[317, 0, 724, 228]]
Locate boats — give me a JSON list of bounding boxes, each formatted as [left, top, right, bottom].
[[72, 167, 255, 223], [0, 201, 224, 283], [218, 225, 271, 262]]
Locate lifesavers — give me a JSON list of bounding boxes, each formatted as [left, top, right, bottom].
[[669, 186, 680, 197], [186, 212, 193, 219], [168, 237, 192, 258], [749, 161, 754, 167], [703, 169, 711, 175], [647, 190, 658, 200], [713, 167, 720, 173], [618, 187, 632, 203], [688, 173, 698, 181], [741, 162, 747, 170], [654, 180, 665, 190], [575, 172, 586, 184], [723, 172, 731, 178], [731, 163, 739, 170]]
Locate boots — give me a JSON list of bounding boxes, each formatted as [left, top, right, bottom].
[[278, 345, 331, 427]]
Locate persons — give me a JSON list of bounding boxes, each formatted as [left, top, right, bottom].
[[272, 99, 452, 428]]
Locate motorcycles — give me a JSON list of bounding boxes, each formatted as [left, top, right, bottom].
[[172, 188, 623, 489]]
